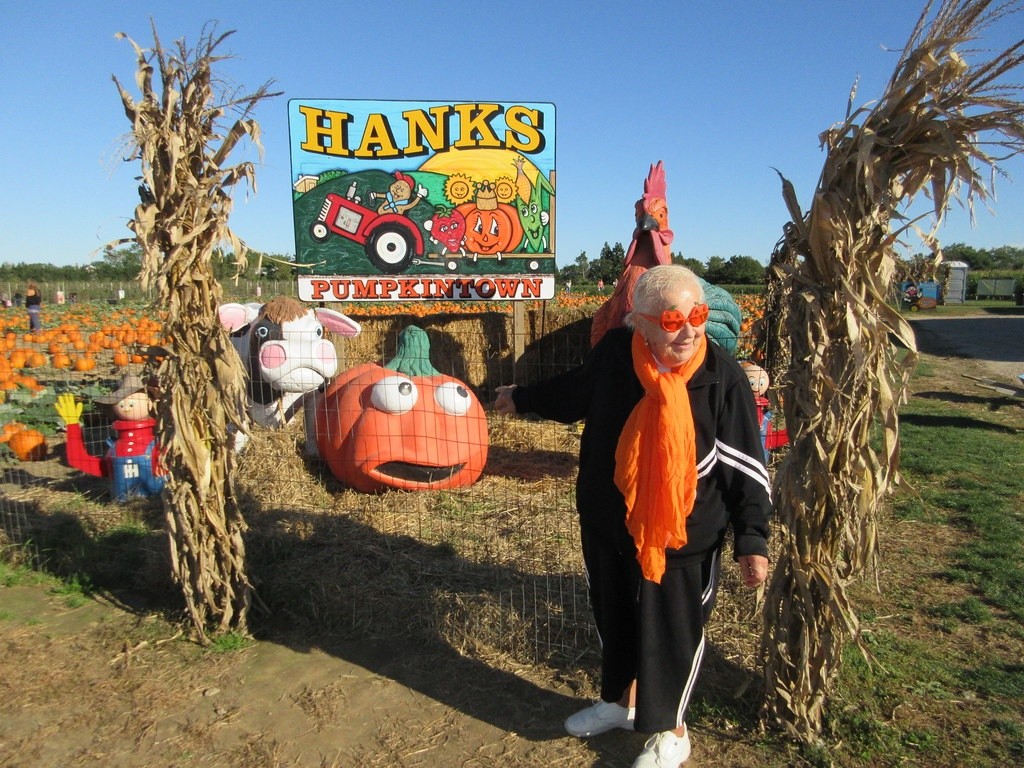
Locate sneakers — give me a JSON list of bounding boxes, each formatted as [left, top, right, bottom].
[[631, 721, 691, 768], [563, 698, 636, 737]]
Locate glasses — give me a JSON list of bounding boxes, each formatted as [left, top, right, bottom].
[[636, 303, 709, 332]]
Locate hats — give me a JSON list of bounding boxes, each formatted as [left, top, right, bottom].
[[93, 375, 161, 422]]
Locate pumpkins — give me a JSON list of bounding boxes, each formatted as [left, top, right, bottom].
[[339, 302, 514, 315], [523, 291, 612, 309], [733, 294, 766, 368], [0, 292, 174, 461]]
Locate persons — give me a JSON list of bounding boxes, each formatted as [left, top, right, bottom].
[[1, 288, 9, 308], [14, 290, 24, 307], [68, 291, 77, 304], [494, 265, 777, 768], [25, 283, 43, 333], [598, 278, 605, 292], [54, 376, 173, 505], [565, 278, 571, 294], [613, 278, 618, 289]]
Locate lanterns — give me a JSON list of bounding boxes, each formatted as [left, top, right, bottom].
[[319, 325, 489, 490]]
